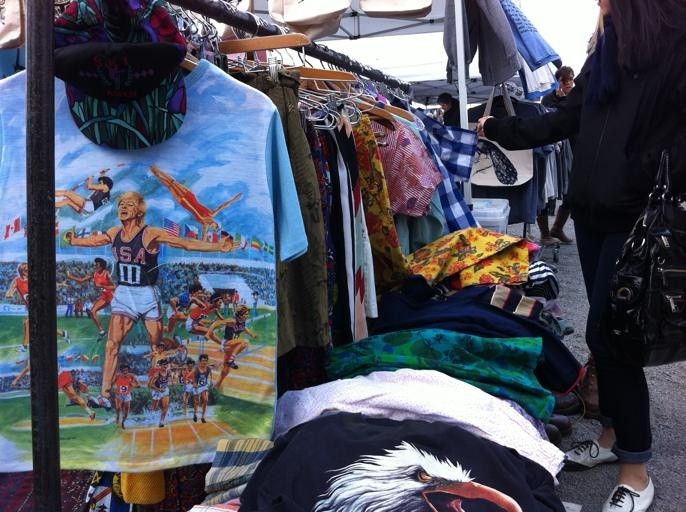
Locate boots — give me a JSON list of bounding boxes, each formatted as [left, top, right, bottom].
[[537, 206, 574, 246], [556, 368, 601, 417]]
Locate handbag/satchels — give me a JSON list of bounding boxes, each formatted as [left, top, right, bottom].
[[607, 149, 685, 369]]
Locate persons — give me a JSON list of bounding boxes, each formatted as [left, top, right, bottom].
[[437, 91, 460, 126], [541, 64, 578, 246], [476, 0, 686, 512]]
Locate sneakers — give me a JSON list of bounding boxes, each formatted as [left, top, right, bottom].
[[562, 438, 619, 472], [601, 476, 654, 512], [224, 361, 238, 371], [87, 394, 113, 410], [97, 330, 106, 342], [62, 330, 71, 345], [14, 346, 28, 351], [90, 413, 207, 430]]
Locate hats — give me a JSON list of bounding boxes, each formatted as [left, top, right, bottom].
[[54, 0, 188, 151]]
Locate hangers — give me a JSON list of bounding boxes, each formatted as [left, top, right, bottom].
[[507, 81, 527, 101], [133, 0, 425, 136]]
